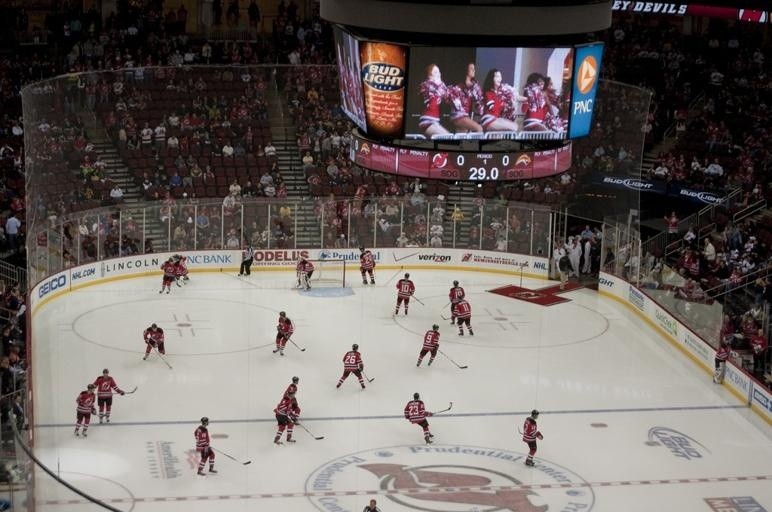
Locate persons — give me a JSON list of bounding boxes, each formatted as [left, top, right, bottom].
[[521, 410, 544, 468], [0, 0, 771, 392], [9, 395, 26, 434], [73, 384, 98, 437], [273, 395, 299, 447], [363, 499, 381, 512], [92, 369, 125, 424], [193, 416, 219, 476], [404, 393, 437, 447], [283, 377, 300, 421]]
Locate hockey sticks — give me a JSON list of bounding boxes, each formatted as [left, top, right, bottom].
[[97, 414, 106, 418], [97, 386, 138, 394], [288, 337, 305, 351], [176, 280, 181, 287], [437, 349, 468, 369], [210, 445, 251, 465], [440, 314, 453, 320], [412, 296, 424, 305], [432, 402, 452, 414], [517, 426, 542, 438], [361, 370, 375, 382], [298, 421, 324, 439]]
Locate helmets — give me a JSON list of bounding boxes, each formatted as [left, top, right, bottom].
[[432, 323, 439, 330], [169, 254, 179, 262], [414, 393, 419, 398], [88, 384, 97, 388], [201, 417, 208, 422], [152, 323, 157, 328], [103, 369, 110, 373], [352, 343, 359, 352], [280, 312, 285, 316], [359, 245, 364, 249], [531, 409, 538, 415]]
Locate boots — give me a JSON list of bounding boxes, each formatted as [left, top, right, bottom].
[[81, 429, 87, 436], [74, 429, 80, 436]]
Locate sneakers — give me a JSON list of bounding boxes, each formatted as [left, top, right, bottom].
[[287, 438, 296, 442], [143, 354, 150, 360], [273, 348, 280, 353], [430, 433, 434, 438], [197, 469, 206, 475], [458, 332, 464, 336], [275, 440, 283, 444], [370, 279, 375, 284], [160, 277, 189, 294], [363, 280, 368, 284], [526, 460, 534, 465], [426, 439, 433, 443], [209, 469, 218, 472], [279, 351, 284, 356]]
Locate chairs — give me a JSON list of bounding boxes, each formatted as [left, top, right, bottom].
[[671, 207, 772, 316], [481, 182, 558, 206], [311, 175, 449, 195], [39, 78, 269, 211]]
